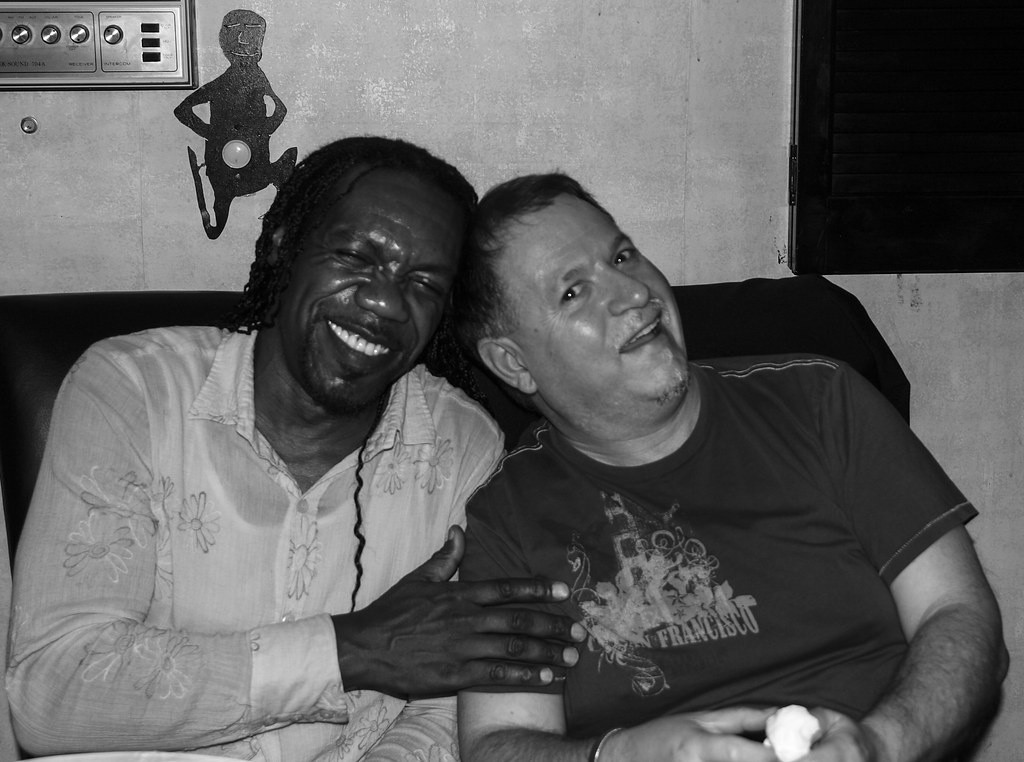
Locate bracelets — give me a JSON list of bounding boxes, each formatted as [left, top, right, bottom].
[[588, 726, 623, 762]]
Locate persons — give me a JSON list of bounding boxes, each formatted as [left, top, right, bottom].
[[452, 174, 1009, 762], [5, 138, 589, 762]]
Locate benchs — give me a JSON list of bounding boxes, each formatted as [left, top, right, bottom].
[[0, 274, 911, 574]]
